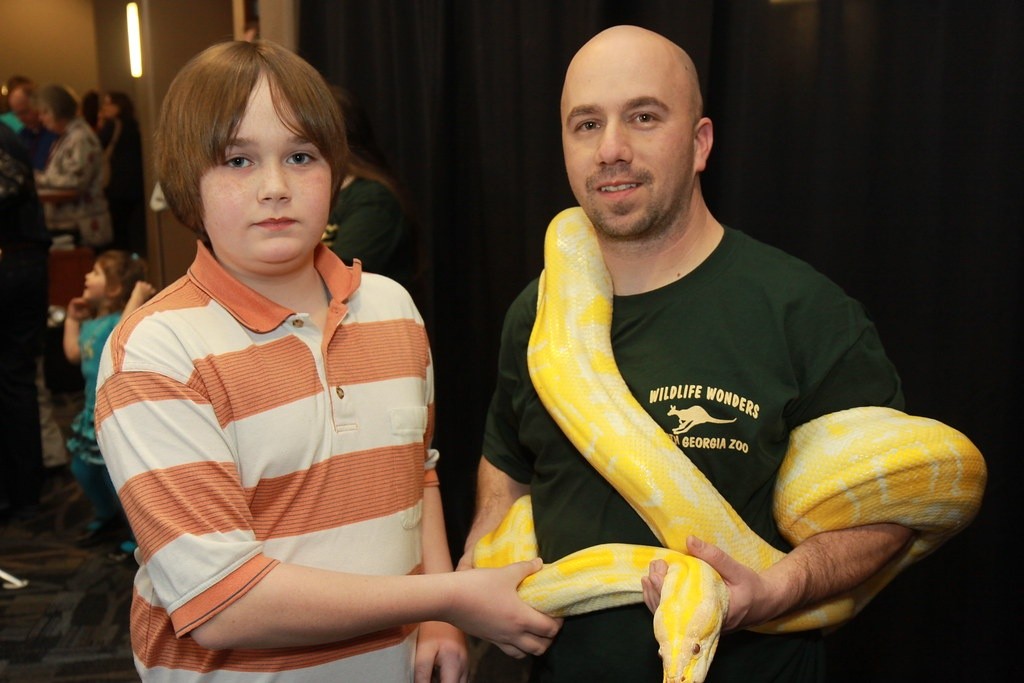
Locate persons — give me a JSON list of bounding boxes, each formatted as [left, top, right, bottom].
[[318, 54, 409, 273], [457, 25, 915, 683], [3, 75, 158, 590], [92, 40, 563, 683]]
